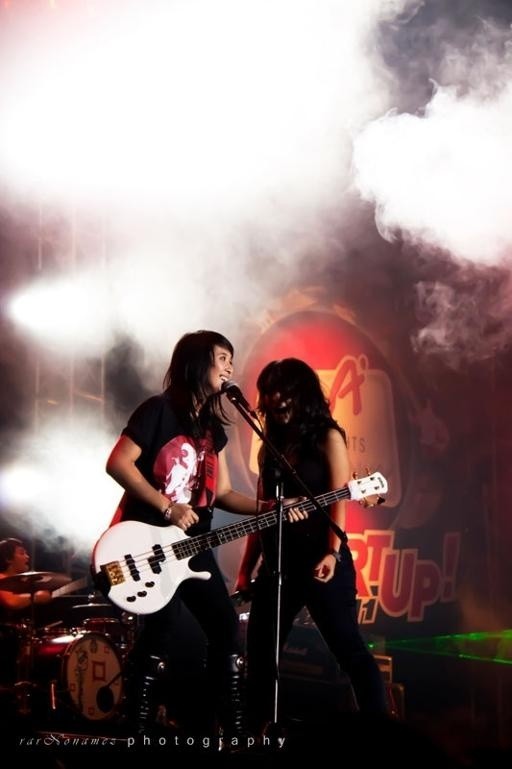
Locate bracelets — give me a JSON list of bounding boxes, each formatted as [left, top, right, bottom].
[[161, 499, 177, 521], [327, 547, 342, 562]]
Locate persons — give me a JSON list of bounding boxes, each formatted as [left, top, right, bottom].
[[235, 357, 388, 723], [0, 537, 74, 686], [106, 331, 309, 731]]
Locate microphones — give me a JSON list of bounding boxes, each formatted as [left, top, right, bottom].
[[221, 380, 258, 420]]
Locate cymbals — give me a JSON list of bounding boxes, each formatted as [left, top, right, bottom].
[[73, 602, 113, 608], [1, 572, 70, 591]]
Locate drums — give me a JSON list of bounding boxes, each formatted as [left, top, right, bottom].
[[34, 628, 127, 722], [82, 618, 121, 640], [1, 624, 35, 668]]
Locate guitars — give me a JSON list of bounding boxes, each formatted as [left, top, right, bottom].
[[91, 467, 389, 616]]
